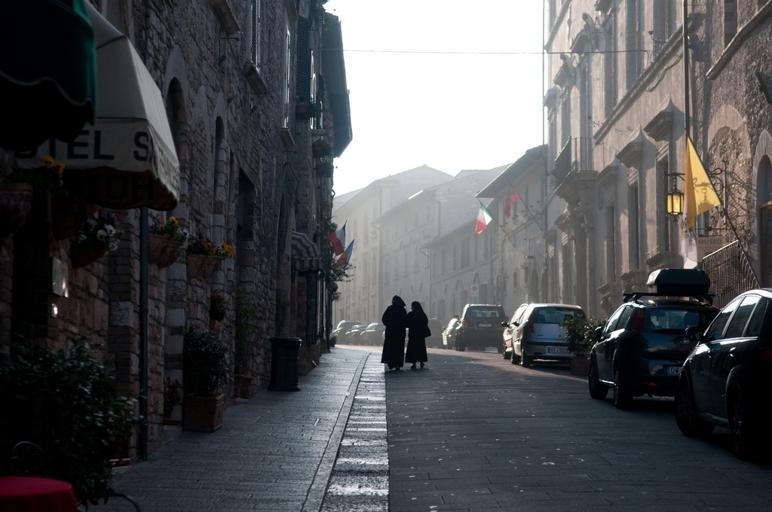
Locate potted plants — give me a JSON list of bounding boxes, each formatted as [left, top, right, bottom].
[[210, 288, 230, 331], [235, 288, 265, 398], [561, 313, 610, 375], [184, 324, 230, 432]]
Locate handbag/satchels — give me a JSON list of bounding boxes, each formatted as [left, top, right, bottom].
[[423, 326, 432, 337]]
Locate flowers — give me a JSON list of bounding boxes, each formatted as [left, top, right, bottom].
[[71, 206, 123, 256], [150, 216, 186, 240], [188, 229, 234, 258]]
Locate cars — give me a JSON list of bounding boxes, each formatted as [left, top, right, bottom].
[[588, 268, 772, 457], [442, 303, 585, 366], [332, 320, 383, 344]]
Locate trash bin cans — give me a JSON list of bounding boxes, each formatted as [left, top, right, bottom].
[[268, 336, 303, 392]]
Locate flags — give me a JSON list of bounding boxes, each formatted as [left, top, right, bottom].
[[503, 189, 521, 223], [474, 202, 493, 234], [330, 222, 345, 256], [679, 135, 722, 257], [334, 242, 354, 271]]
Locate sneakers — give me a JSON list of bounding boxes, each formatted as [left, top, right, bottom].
[[389, 367, 400, 371]]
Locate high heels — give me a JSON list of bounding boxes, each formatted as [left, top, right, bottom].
[[410, 363, 425, 368]]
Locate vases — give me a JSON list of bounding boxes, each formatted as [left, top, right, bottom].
[[188, 254, 225, 276], [150, 233, 184, 266], [68, 239, 105, 267]]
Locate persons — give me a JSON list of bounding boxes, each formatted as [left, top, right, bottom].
[[405, 300, 427, 369], [381, 295, 409, 371], [445, 315, 461, 350]]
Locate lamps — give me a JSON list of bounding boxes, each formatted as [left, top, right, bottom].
[[667, 172, 685, 215]]
